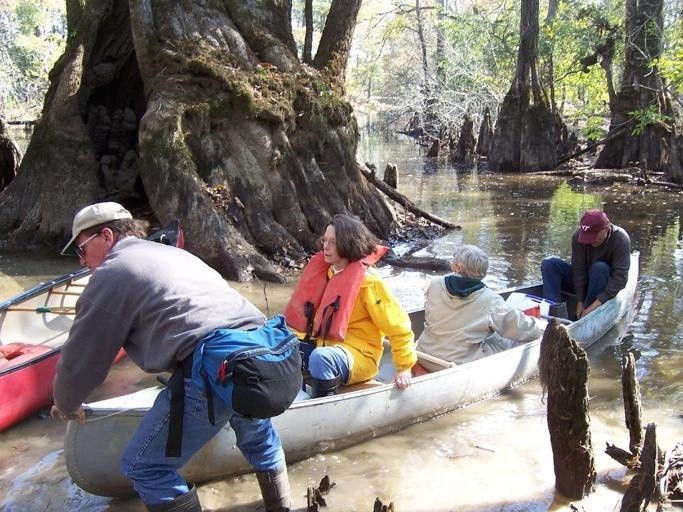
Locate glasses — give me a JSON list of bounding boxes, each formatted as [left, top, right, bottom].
[[73, 233, 98, 257], [320, 237, 337, 246]]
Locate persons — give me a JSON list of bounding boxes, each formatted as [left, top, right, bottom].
[[285, 213, 418, 399], [49, 199, 295, 512], [414, 243, 549, 373], [537, 208, 632, 320]]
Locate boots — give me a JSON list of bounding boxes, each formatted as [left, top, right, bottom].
[[145, 482, 203, 512], [255, 462, 290, 511], [310, 372, 341, 398]]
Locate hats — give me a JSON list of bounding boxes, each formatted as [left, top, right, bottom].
[[578, 209, 608, 244], [61, 202, 133, 256]]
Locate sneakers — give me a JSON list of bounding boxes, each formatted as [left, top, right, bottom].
[[548, 302, 568, 325]]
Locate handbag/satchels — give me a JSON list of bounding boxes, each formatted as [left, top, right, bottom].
[[192, 314, 303, 418]]
